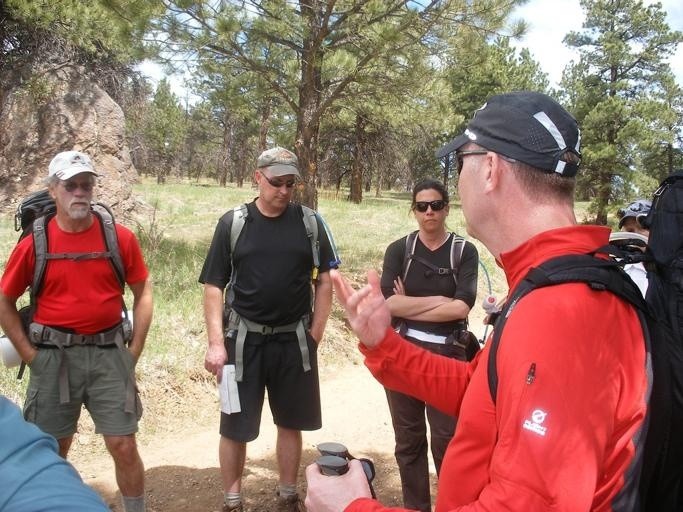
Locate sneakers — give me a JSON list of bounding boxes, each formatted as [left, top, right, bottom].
[[276, 491, 301, 511], [222, 502, 243, 512]]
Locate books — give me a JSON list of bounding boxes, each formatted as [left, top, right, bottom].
[[217, 364, 241, 415]]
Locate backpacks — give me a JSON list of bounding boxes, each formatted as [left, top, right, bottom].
[[487, 174, 683, 512], [15, 189, 128, 348]]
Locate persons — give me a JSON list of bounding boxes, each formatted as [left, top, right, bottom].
[[198, 148, 341, 511], [1, 151, 155, 512], [0, 395, 113, 512], [303, 88, 653, 511], [381, 178, 479, 510], [616, 198, 657, 254]]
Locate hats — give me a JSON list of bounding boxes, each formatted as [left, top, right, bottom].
[[436, 92, 582, 177], [616, 199, 653, 230], [257, 147, 299, 177], [49, 151, 98, 181]]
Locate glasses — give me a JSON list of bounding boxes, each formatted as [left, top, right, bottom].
[[455, 150, 518, 174], [260, 171, 294, 187], [65, 181, 92, 191], [415, 200, 444, 212], [615, 201, 650, 217]]
